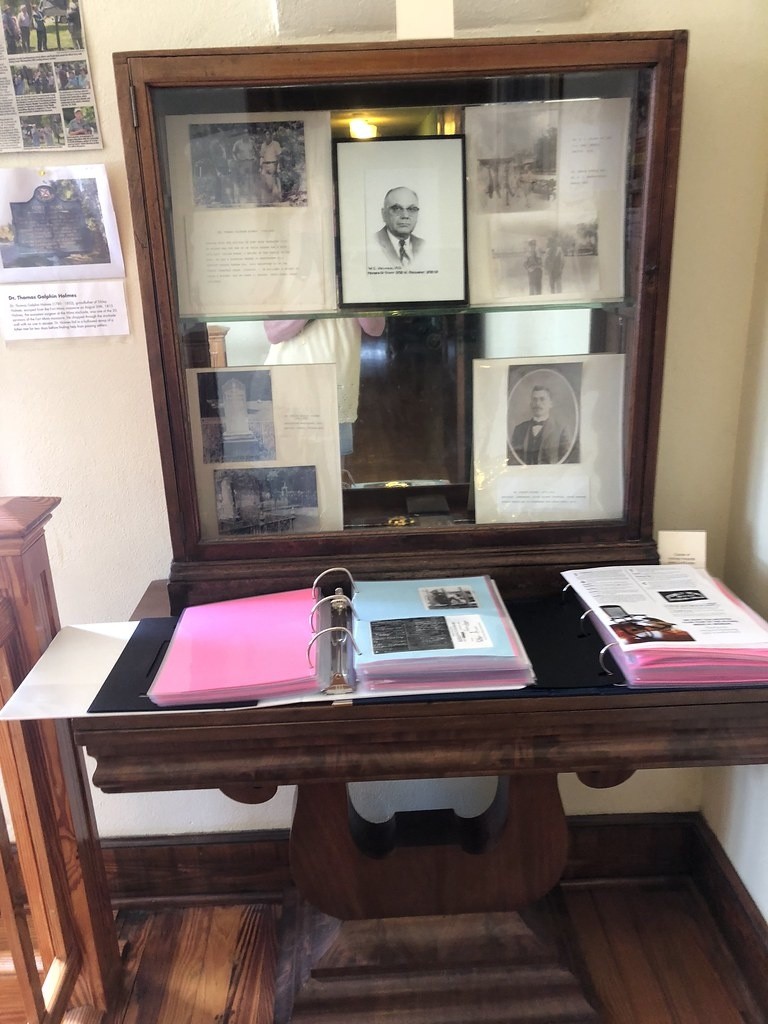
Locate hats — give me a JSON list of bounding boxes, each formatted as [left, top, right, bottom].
[[74, 108, 82, 114], [70, 2, 77, 10], [3, 4, 10, 11]]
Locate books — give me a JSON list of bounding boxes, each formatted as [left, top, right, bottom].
[[86, 567, 768, 712]]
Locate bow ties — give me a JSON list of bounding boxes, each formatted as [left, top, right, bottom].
[[533, 421, 547, 426]]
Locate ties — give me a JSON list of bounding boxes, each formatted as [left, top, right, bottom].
[[399, 239, 411, 266]]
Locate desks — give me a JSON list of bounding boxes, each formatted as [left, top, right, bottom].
[[72, 579, 768, 1024]]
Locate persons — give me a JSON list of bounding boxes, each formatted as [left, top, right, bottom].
[[68, 3, 84, 50], [432, 591, 463, 603], [32, 123, 56, 145], [68, 109, 93, 135], [14, 68, 53, 93], [264, 317, 386, 455], [526, 238, 566, 297], [376, 186, 428, 267], [212, 127, 296, 199], [2, 3, 47, 52], [59, 63, 88, 88], [512, 385, 570, 463]]
